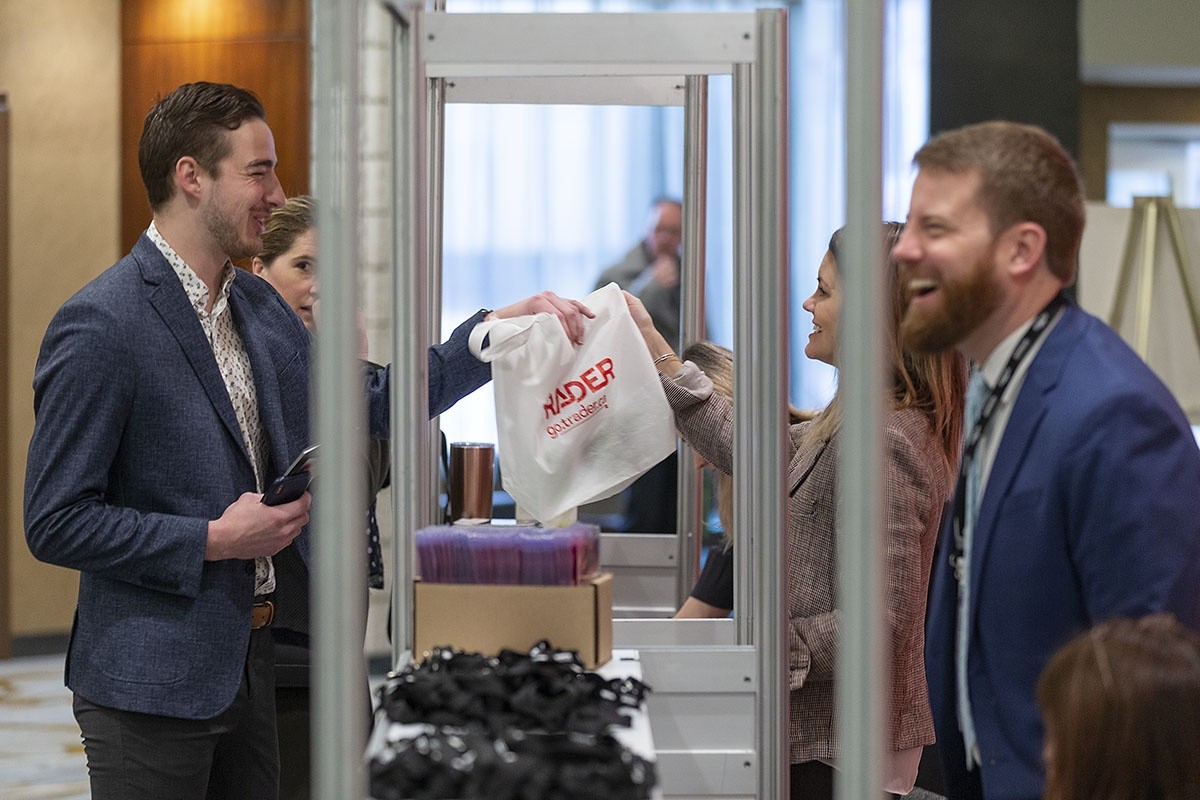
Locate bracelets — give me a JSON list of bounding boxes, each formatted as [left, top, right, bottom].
[[654, 352, 675, 365]]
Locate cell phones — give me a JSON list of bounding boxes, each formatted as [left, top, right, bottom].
[[261, 471, 312, 507]]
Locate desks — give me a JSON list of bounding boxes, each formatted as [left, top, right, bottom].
[[362, 649, 658, 800]]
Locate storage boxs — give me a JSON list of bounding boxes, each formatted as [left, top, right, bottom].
[[413, 573, 614, 672]]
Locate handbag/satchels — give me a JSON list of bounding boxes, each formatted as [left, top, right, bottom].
[[469, 282, 679, 523]]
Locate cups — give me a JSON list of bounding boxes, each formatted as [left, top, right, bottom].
[[516, 502, 579, 528], [450, 442, 496, 526]]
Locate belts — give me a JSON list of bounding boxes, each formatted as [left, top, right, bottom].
[[250, 600, 278, 629]]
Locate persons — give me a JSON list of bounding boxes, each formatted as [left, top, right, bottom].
[[24, 82, 595, 800], [891, 123, 1199, 800], [1038, 613, 1200, 800], [620, 221, 972, 800], [592, 197, 682, 535], [251, 195, 390, 800], [672, 341, 820, 619]]
